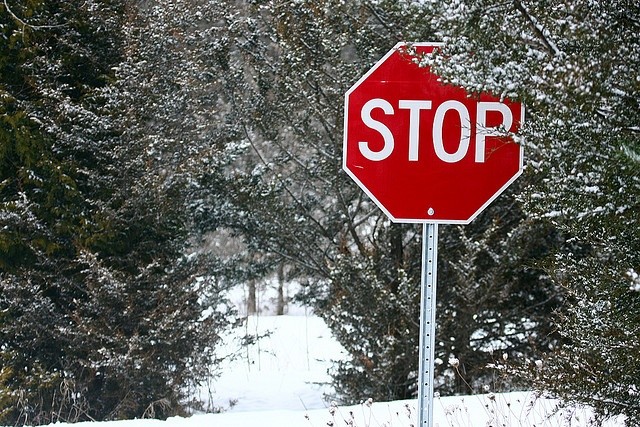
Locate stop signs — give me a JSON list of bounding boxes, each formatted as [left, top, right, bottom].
[[342, 41, 526, 224]]
